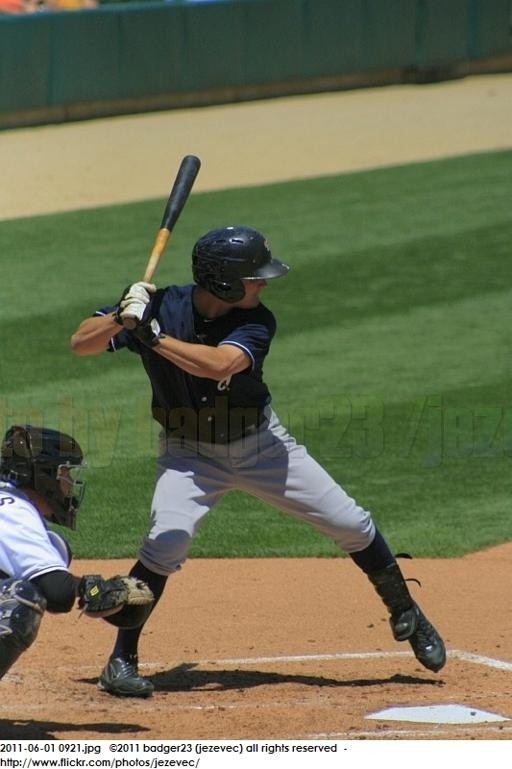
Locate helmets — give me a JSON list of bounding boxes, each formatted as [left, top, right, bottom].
[[1, 424, 87, 529], [192, 226, 290, 302]]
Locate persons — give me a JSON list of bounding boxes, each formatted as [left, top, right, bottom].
[[69, 226, 446, 695], [0, 424, 155, 685]]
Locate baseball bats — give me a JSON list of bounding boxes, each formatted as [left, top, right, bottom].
[[122, 155, 200, 331]]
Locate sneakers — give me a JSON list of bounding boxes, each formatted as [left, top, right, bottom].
[[389, 602, 446, 670], [99, 654, 154, 696]]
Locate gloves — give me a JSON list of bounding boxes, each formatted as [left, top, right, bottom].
[[113, 282, 165, 344]]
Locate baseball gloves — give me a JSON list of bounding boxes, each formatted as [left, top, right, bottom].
[[79, 574, 154, 631]]
